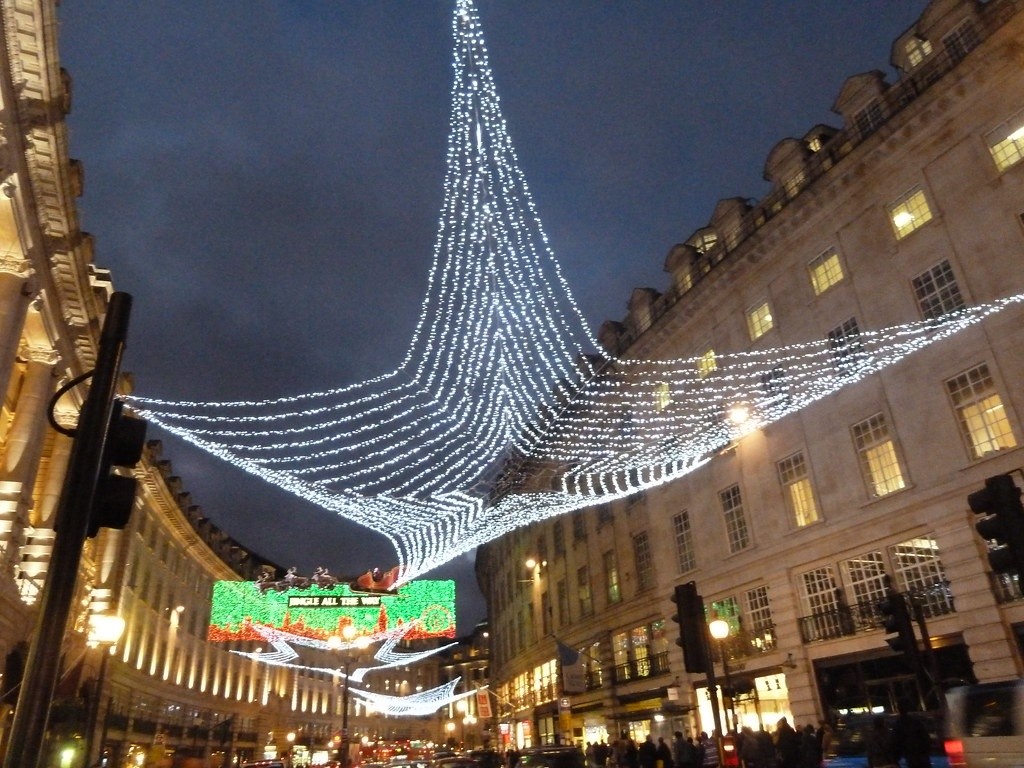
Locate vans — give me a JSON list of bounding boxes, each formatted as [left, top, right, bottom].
[[944, 678, 1024, 768]]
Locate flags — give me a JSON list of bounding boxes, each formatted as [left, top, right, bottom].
[[475, 685, 492, 718], [558, 642, 586, 692]]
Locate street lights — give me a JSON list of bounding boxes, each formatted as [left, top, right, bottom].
[[463, 713, 477, 754], [79, 598, 125, 768], [327, 621, 370, 768], [447, 722, 456, 751], [708, 608, 744, 768]]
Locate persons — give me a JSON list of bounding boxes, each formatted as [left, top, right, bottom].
[[608, 730, 719, 768], [569, 739, 608, 768], [726, 717, 834, 768], [907, 587, 931, 618], [478, 748, 516, 768]]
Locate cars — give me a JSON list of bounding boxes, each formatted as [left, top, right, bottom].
[[240, 750, 505, 768], [514, 746, 584, 768], [819, 714, 950, 768]]
